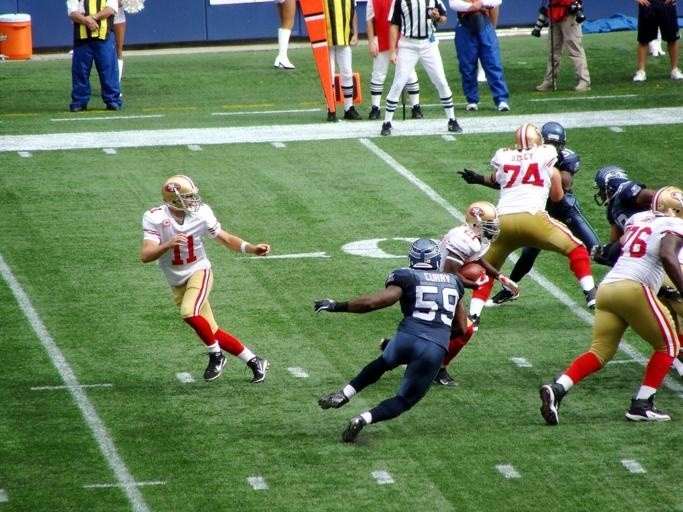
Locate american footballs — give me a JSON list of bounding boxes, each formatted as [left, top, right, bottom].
[[459, 263, 486, 281]]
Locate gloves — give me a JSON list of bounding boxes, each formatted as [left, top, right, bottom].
[[313, 298, 348, 312], [475, 273, 520, 296]]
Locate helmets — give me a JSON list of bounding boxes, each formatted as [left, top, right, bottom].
[[163, 175, 201, 212]]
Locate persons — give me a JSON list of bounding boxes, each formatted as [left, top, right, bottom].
[[309, 233, 468, 445], [323, 0, 509, 136], [139, 173, 273, 387], [377, 202, 520, 386], [271, 0, 298, 72], [464, 121, 599, 332], [111, 0, 127, 83], [65, 1, 121, 110], [590, 164, 682, 376], [536, 0, 591, 92], [456, 123, 605, 308], [538, 185, 683, 427], [633, 0, 683, 82]]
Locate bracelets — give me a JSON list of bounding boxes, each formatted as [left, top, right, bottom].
[[239, 240, 251, 253], [91, 15, 96, 21]]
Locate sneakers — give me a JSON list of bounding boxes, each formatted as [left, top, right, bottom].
[[381, 122, 392, 136]]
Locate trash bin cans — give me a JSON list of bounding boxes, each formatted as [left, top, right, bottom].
[[0, 13, 32, 59]]
